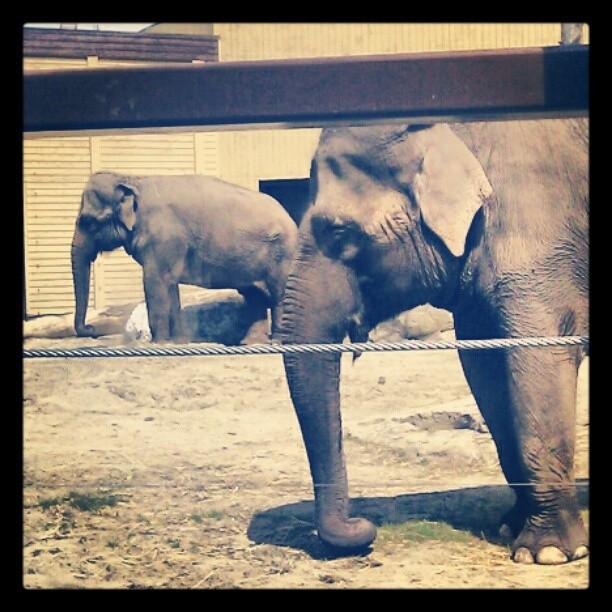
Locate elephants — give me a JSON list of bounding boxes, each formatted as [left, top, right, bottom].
[[280, 115, 590, 567], [70, 170, 299, 345]]
